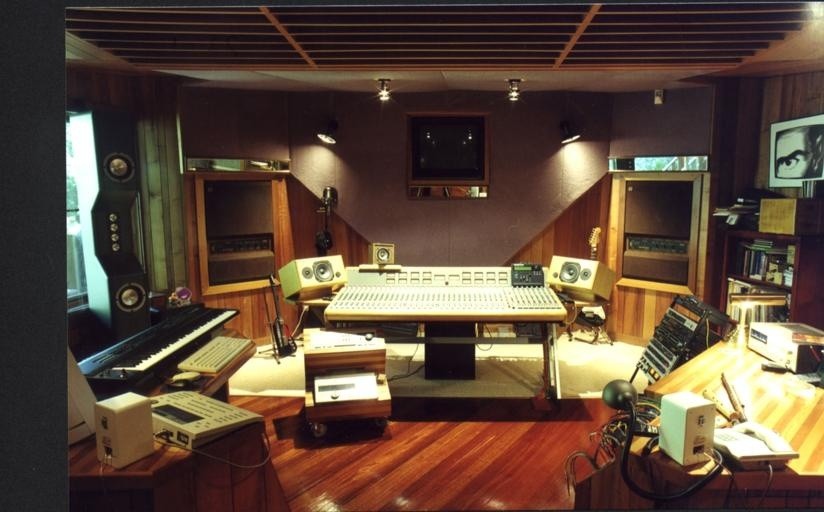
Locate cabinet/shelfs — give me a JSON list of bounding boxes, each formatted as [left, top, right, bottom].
[[719, 230, 824, 326]]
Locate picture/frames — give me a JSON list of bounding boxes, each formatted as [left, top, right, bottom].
[[403, 109, 489, 202]]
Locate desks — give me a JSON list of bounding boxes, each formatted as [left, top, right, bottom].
[[647, 325, 824, 511]]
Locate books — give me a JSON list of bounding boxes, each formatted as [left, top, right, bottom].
[[724, 239, 793, 327]]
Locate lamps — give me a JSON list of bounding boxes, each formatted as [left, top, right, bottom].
[[315, 119, 338, 146], [556, 119, 583, 146], [727, 293, 787, 364], [376, 78, 391, 102], [504, 78, 525, 103]]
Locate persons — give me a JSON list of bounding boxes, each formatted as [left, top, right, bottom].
[[776, 125, 824, 179]]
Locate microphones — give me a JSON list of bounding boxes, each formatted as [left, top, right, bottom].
[[322, 188, 337, 207]]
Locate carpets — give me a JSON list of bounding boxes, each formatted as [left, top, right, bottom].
[[223, 302, 645, 400]]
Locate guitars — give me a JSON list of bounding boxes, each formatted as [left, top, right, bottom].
[[588, 227, 603, 261], [268, 271, 297, 357]]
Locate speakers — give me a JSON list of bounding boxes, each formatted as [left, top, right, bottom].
[[69, 105, 152, 344], [621, 181, 693, 285], [368, 242, 395, 265], [278, 254, 349, 302], [657, 390, 718, 468], [93, 392, 155, 471], [423, 322, 476, 382], [201, 179, 276, 286], [546, 255, 617, 301]]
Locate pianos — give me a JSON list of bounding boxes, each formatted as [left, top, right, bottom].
[[77, 305, 240, 382]]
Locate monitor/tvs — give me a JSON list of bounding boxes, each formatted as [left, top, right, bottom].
[[407, 112, 489, 186]]
